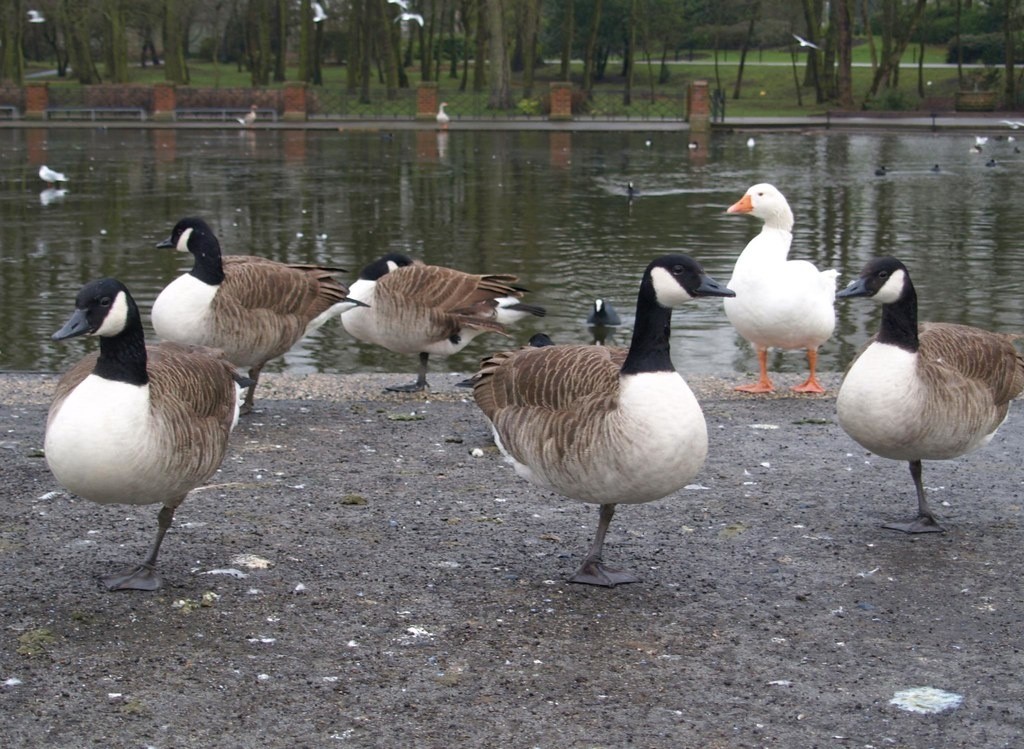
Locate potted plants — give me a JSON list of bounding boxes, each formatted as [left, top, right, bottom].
[[954, 65, 1001, 111]]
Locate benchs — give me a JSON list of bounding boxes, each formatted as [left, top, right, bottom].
[[43, 105, 148, 122], [173, 108, 279, 122], [0, 105, 18, 118]]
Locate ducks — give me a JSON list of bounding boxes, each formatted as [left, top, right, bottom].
[[150, 217, 369, 417], [723, 182, 840, 393], [237, 106, 259, 129], [930, 163, 940, 173], [875, 167, 886, 177], [970, 135, 1021, 169], [622, 136, 757, 198], [436, 102, 450, 128], [836, 255, 1024, 533], [454, 332, 556, 387], [38, 165, 68, 184], [469, 253, 736, 586], [340, 253, 546, 394], [587, 297, 623, 325], [42, 280, 261, 592]]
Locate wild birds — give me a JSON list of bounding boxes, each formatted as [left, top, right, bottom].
[[1002, 120, 1024, 130], [388, 0, 424, 27], [312, 4, 328, 21], [793, 33, 819, 50], [27, 9, 46, 24]]
